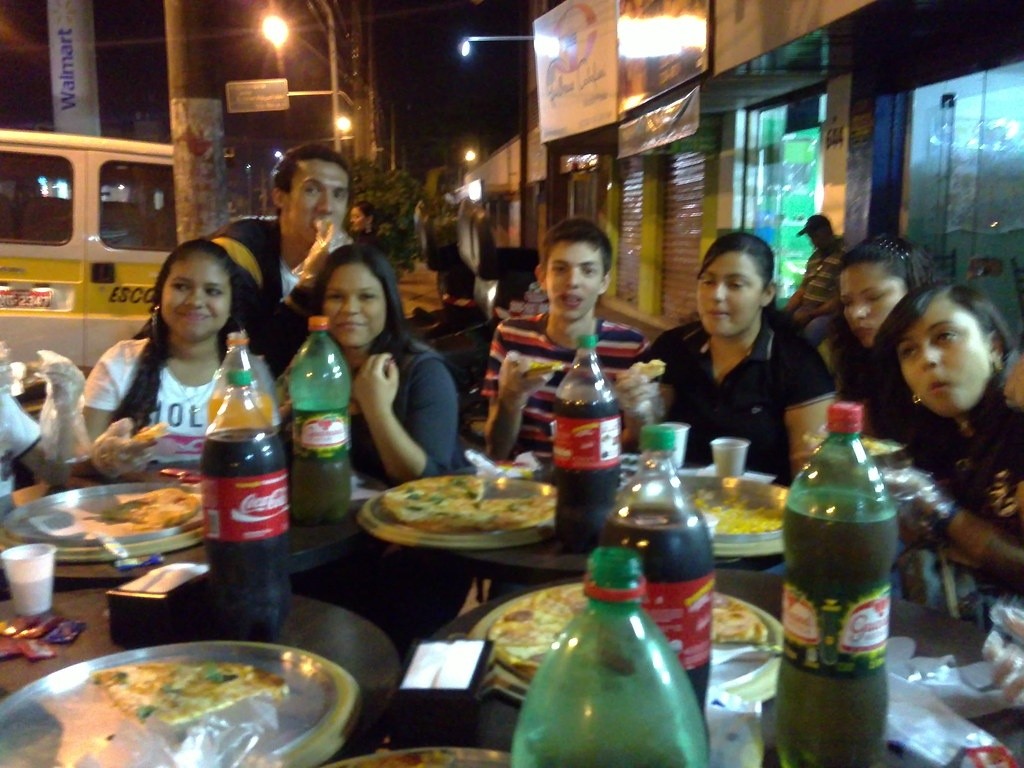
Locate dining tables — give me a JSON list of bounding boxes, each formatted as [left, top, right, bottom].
[[0, 460, 1024, 768]]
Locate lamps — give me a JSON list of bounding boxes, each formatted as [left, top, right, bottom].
[[457, 36, 534, 56]]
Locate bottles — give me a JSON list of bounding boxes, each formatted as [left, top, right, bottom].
[[207, 330, 281, 460], [512, 546, 713, 768], [769, 400, 896, 768], [202, 372, 293, 635], [551, 332, 622, 551], [286, 316, 352, 523], [601, 425, 714, 730]]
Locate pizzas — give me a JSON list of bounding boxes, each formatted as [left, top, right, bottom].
[[640, 359, 665, 380], [383, 475, 554, 529], [132, 422, 168, 438], [522, 358, 561, 374], [89, 660, 287, 724], [101, 488, 200, 527], [487, 585, 769, 681]]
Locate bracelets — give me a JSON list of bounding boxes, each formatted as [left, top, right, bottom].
[[497, 399, 527, 412], [932, 502, 967, 534]]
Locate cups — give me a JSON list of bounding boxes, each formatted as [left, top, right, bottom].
[[710, 437, 752, 478], [656, 423, 691, 469], [0, 543, 57, 616]]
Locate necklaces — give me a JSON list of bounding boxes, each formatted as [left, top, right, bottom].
[[954, 426, 975, 472]]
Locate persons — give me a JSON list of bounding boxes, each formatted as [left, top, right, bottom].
[[878, 466, 1024, 594], [834, 233, 933, 440], [783, 215, 848, 350], [873, 279, 1013, 515], [480, 217, 651, 605], [204, 142, 355, 313], [350, 201, 377, 238], [79, 237, 292, 479], [316, 244, 474, 661], [630, 231, 835, 488], [0, 360, 84, 498]]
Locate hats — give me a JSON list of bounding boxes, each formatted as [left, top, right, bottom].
[[797, 215, 831, 237]]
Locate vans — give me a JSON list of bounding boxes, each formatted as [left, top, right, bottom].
[[0, 129, 178, 492]]
